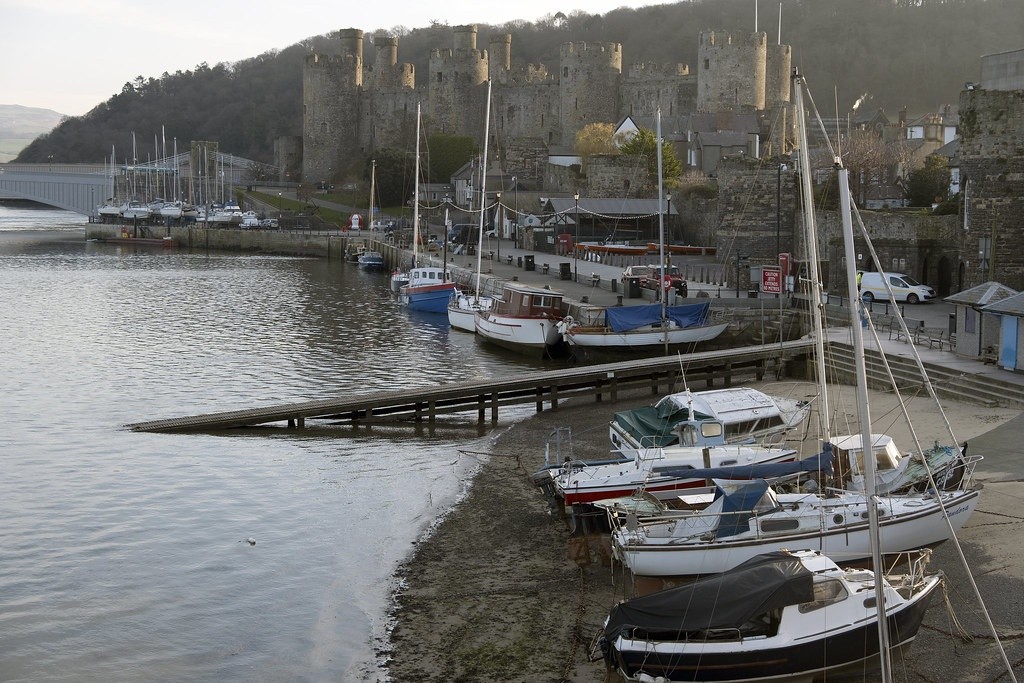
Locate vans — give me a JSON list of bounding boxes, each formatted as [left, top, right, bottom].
[[449, 224, 479, 241], [857, 269, 937, 305]]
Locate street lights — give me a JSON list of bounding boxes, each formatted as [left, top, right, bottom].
[[573, 192, 580, 282], [496, 191, 501, 261], [665, 191, 671, 275], [279, 192, 282, 220], [48, 153, 54, 166], [445, 191, 448, 210], [512, 177, 517, 248]]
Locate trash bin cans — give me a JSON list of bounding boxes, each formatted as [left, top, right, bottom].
[[949, 313, 955, 340], [466, 242, 475, 255], [559, 263, 571, 280], [524, 255, 535, 271], [430, 235, 437, 240], [624, 276, 640, 298]]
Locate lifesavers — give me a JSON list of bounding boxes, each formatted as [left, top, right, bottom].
[[659, 275, 672, 291]]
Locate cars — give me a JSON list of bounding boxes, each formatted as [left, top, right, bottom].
[[369, 220, 396, 232], [473, 282, 563, 359], [622, 265, 654, 289], [240, 210, 278, 229], [486, 228, 497, 239]]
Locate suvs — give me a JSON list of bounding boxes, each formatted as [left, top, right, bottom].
[[647, 263, 687, 299]]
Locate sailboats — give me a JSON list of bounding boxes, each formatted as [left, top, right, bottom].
[[358, 158, 385, 267], [564, 106, 732, 348], [95, 121, 243, 223], [602, 69, 986, 579], [388, 103, 423, 290], [447, 75, 493, 332]]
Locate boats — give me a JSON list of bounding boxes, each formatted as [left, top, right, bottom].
[[598, 548, 943, 682], [400, 206, 455, 313], [529, 428, 800, 508], [345, 240, 365, 261], [608, 385, 812, 458]]
